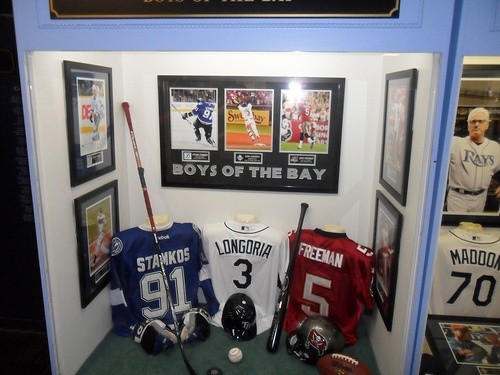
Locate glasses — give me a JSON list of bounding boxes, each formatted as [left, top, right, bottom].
[[469, 120, 487, 124]]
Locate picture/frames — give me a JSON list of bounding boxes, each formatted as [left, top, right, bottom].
[[63, 60, 115, 187], [157, 75, 346, 194], [377, 68, 418, 206], [372, 190, 403, 333], [74, 180, 119, 308], [441, 65, 500, 228]]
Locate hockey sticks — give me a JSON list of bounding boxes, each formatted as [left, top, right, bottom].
[[171, 103, 192, 125], [120, 101, 223, 375]]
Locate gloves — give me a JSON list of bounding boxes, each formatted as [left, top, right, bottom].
[[132, 319, 179, 355], [179, 308, 211, 344], [182, 113, 188, 120]]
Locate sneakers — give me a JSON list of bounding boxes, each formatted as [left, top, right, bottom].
[[195, 134, 201, 143], [210, 140, 216, 148]]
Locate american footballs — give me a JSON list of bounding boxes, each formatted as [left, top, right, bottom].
[[315, 352, 373, 375]]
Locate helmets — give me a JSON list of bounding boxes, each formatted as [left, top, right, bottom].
[[222, 293, 257, 342], [286, 315, 345, 367], [242, 102, 247, 107]]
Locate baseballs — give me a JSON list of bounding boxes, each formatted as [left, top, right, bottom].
[[228, 347, 244, 364]]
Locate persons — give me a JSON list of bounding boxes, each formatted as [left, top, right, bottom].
[[180, 96, 215, 147], [171, 89, 216, 103], [88, 86, 107, 142], [292, 99, 315, 150], [225, 89, 272, 107], [441, 322, 500, 368], [281, 89, 330, 122], [92, 207, 113, 267], [76, 79, 106, 96], [443, 107, 500, 214], [232, 99, 260, 143]]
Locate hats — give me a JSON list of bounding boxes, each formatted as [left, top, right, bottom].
[[451, 325, 468, 331], [476, 327, 498, 335]]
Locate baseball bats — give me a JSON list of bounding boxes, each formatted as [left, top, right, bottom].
[[264, 202, 309, 354]]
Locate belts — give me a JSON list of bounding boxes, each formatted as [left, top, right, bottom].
[[451, 188, 485, 196]]
